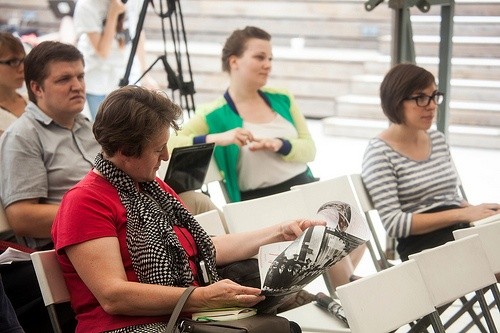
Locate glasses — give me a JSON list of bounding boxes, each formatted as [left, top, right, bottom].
[[0, 55, 24, 67], [403, 92, 445, 106]]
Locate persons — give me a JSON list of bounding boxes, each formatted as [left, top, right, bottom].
[[361, 63, 500, 263], [167, 25, 366, 298], [0, 0, 153, 252], [51, 86, 325, 333]]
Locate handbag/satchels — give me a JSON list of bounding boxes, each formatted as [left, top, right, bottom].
[[165, 285, 292, 332]]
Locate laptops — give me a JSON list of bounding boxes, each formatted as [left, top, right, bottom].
[[163, 142, 216, 194]]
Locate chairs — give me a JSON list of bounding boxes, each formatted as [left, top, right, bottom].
[[30, 173, 500, 333]]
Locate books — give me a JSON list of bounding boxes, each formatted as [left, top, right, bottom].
[[192, 308, 257, 321]]
